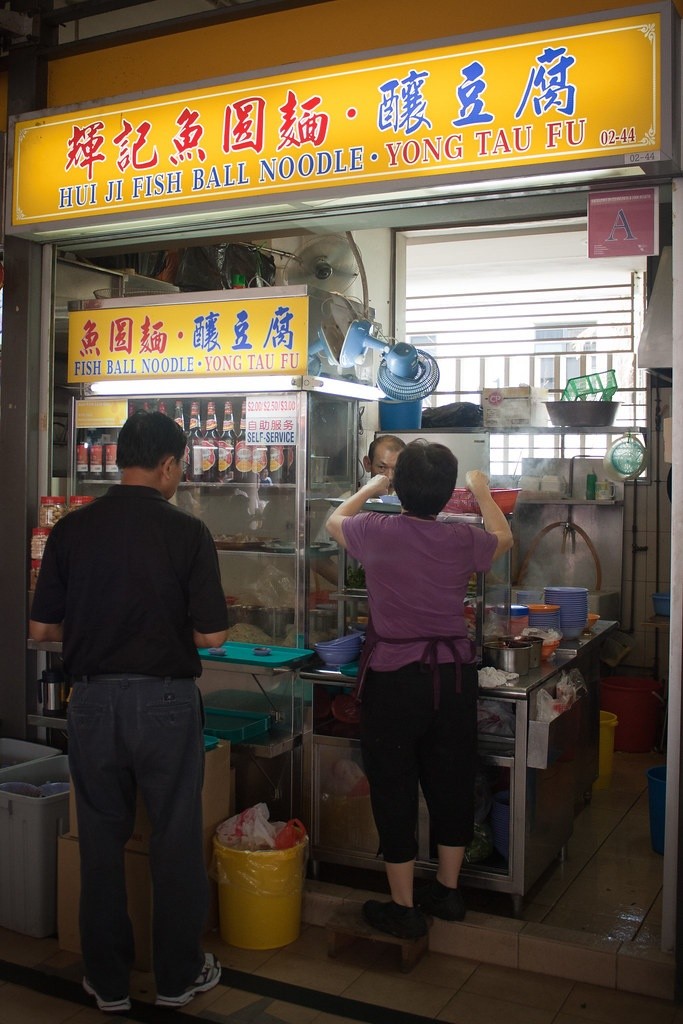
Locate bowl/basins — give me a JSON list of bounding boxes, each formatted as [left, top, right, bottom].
[[314, 632, 364, 666], [650, 591, 670, 616], [516, 587, 601, 664], [450, 487, 522, 515], [482, 634, 544, 677], [228, 602, 337, 640], [540, 400, 622, 428], [38, 783, 70, 796], [1, 782, 42, 799], [486, 800, 511, 865]]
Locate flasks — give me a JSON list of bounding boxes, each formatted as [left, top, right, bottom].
[[35, 665, 63, 718]]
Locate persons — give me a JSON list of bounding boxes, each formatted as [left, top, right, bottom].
[[309, 435, 406, 616], [326, 441, 513, 941], [30, 411, 231, 1012]]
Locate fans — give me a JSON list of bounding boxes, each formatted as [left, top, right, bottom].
[[338, 319, 441, 403], [283, 235, 362, 295], [306, 319, 345, 377]]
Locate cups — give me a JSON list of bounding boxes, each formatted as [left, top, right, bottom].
[[310, 457, 328, 483], [593, 481, 616, 500]]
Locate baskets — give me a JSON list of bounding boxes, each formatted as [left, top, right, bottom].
[[602, 434, 648, 482], [562, 368, 617, 401], [441, 487, 521, 515]]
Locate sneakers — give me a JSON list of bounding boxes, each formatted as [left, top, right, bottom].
[[153, 951, 222, 1007], [83, 975, 132, 1012]]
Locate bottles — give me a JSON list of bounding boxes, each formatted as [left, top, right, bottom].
[[73, 390, 297, 481], [29, 495, 94, 588], [586, 472, 597, 500], [495, 604, 528, 639]]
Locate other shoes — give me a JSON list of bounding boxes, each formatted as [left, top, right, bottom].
[[360, 898, 428, 940], [413, 874, 466, 922]]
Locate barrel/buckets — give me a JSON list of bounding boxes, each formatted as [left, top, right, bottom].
[[597, 672, 667, 753], [376, 387, 423, 431], [212, 833, 308, 949], [591, 709, 619, 792], [646, 763, 667, 855]]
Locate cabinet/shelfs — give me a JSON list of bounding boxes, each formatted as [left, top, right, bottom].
[[27, 391, 359, 757], [370, 426, 646, 507]]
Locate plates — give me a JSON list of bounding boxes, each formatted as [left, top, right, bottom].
[[211, 532, 333, 552]]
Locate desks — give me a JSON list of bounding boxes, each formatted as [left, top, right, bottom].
[[640, 613, 670, 754]]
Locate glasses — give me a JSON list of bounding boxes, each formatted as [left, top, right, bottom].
[[173, 457, 190, 471]]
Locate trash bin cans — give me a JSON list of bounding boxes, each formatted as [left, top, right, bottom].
[[213, 836, 309, 952]]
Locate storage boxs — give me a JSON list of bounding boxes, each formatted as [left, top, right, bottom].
[[379, 397, 423, 429], [483, 387, 548, 427], [58, 740, 235, 973]]
